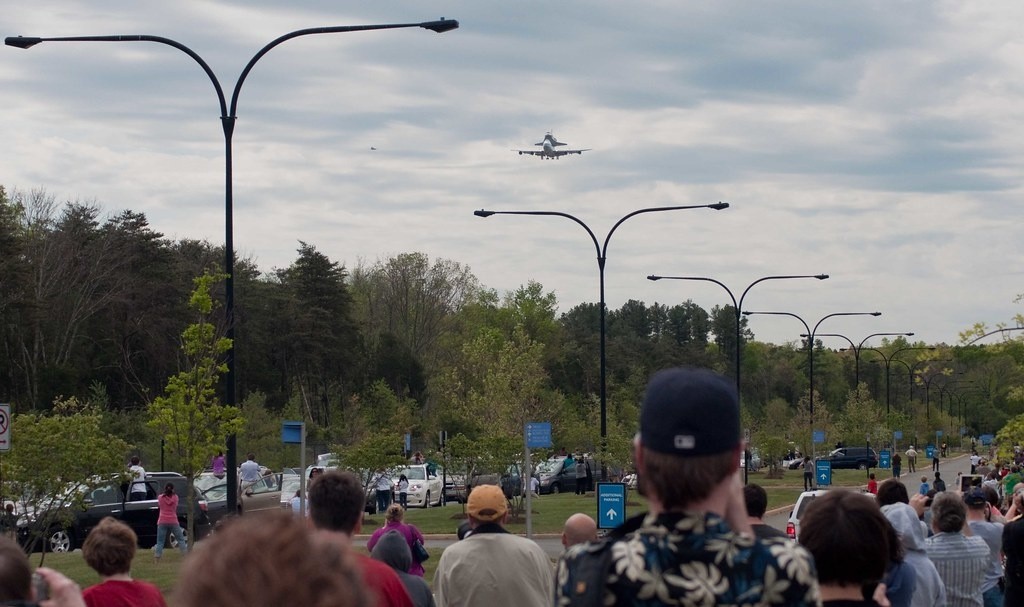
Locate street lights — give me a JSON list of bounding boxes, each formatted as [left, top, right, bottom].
[[4, 16, 458, 545], [644, 269, 825, 495], [739, 308, 871, 500], [798, 331, 970, 496], [473, 197, 733, 500]]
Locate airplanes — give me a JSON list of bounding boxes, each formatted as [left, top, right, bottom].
[[514, 135, 589, 161]]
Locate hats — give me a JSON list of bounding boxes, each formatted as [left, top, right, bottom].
[[127, 461, 133, 468], [467, 485, 507, 520], [964, 486, 986, 505], [641, 369, 740, 457]]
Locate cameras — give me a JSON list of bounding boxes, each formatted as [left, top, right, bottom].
[[31, 573, 50, 601]]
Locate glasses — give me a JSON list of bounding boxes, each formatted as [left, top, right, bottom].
[[312, 471, 318, 473]]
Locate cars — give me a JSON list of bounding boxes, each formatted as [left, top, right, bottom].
[[440, 460, 502, 502], [391, 463, 447, 508], [814, 447, 879, 469], [281, 474, 315, 510], [533, 454, 622, 497], [13, 469, 211, 549], [206, 472, 296, 525]]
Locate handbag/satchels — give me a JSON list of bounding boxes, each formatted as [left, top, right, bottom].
[[408, 523, 430, 564]]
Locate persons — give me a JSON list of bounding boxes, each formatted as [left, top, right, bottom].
[[0, 444, 1024, 607], [557, 365, 822, 607], [432, 484, 557, 607]]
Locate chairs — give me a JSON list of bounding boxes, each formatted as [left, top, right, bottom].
[[93, 489, 117, 504]]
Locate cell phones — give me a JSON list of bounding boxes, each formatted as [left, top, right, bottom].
[[925, 499, 933, 507]]
